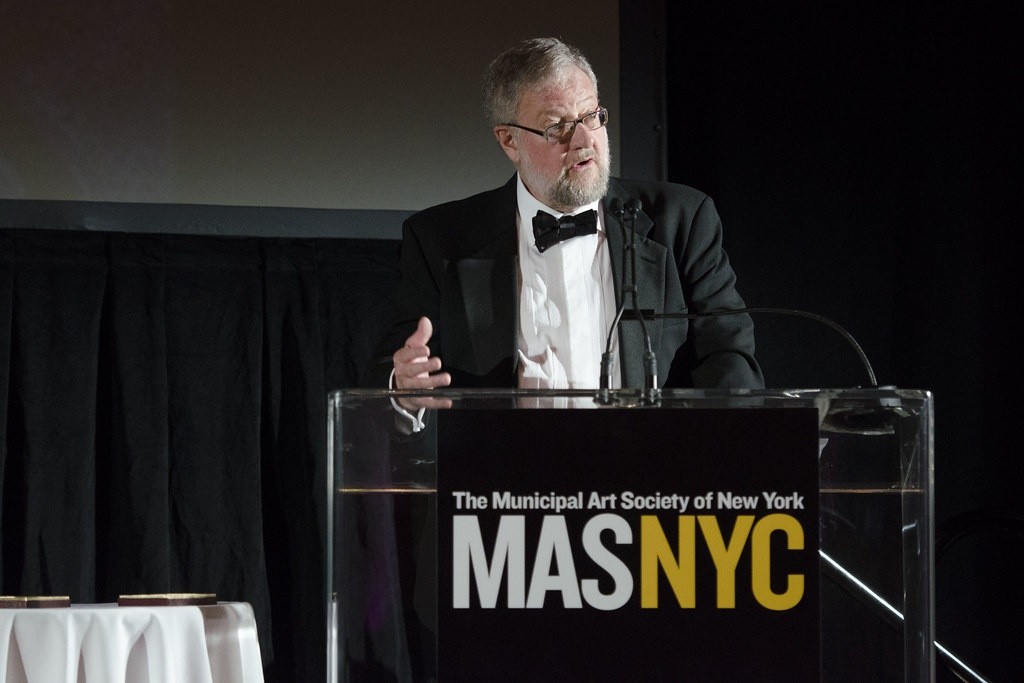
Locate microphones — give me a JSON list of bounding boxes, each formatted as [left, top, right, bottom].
[[598, 197, 878, 404]]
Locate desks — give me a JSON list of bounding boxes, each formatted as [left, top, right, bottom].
[[0, 600, 266, 683]]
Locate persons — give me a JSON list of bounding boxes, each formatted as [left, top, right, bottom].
[[362, 38, 771, 485]]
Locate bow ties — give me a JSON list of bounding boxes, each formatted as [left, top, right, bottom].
[[532, 209, 599, 253]]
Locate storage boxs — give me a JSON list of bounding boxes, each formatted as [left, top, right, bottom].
[[0, 596, 71, 608], [118, 593, 217, 606]]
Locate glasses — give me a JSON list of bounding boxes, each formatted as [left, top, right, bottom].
[[496, 104, 609, 144]]
